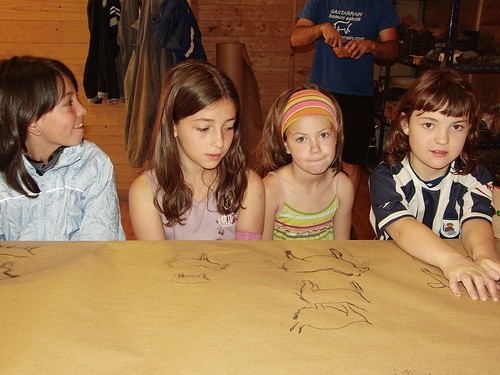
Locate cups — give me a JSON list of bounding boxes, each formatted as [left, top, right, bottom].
[[333, 46, 350, 59]]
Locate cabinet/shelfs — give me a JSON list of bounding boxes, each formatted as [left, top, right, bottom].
[[371, 0, 500, 179]]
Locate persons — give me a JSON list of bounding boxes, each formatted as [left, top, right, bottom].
[[291, 0, 399, 161], [0, 55, 127, 241], [366, 68, 500, 301], [252, 83, 355, 241], [128, 60, 266, 240]]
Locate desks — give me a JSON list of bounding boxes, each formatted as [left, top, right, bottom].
[[1, 241, 500, 375]]
